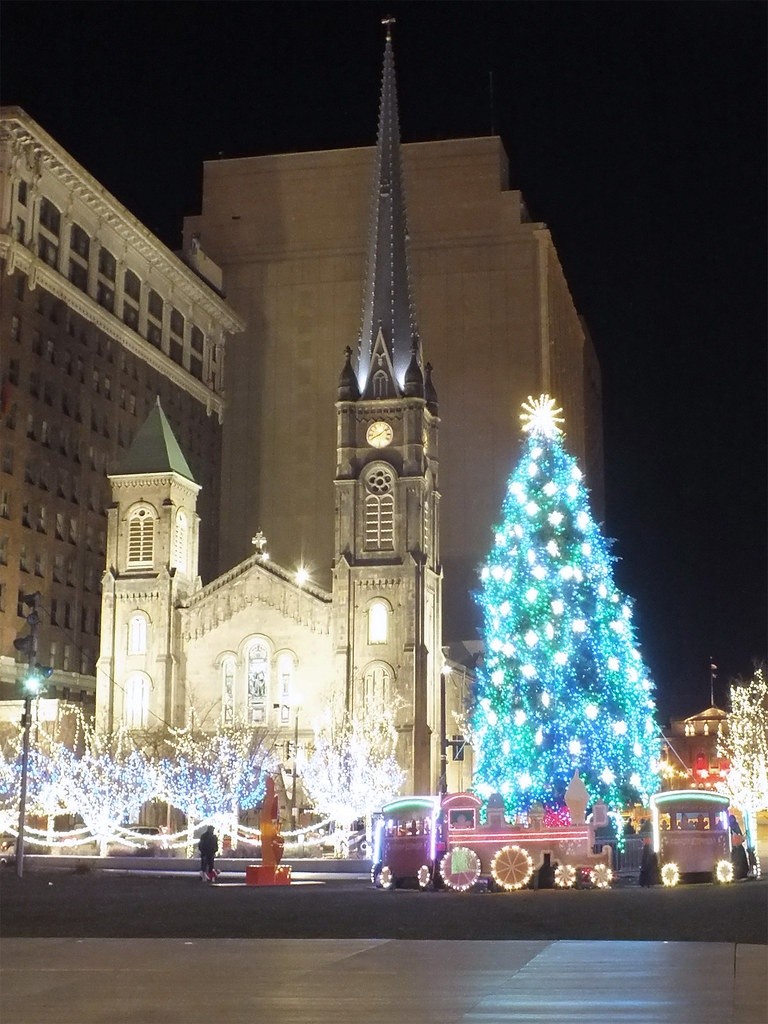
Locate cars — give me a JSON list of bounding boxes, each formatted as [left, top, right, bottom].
[[59, 821, 97, 854], [0, 825, 33, 853]]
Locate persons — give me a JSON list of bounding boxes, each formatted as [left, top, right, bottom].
[[199, 827, 218, 880], [694, 814, 708, 830], [624, 818, 653, 835], [729, 815, 745, 842], [678, 814, 694, 830]]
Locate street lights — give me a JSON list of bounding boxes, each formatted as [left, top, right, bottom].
[[439, 664, 453, 797], [273, 703, 299, 842], [15, 591, 54, 878]]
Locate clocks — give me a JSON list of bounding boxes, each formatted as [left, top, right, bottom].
[[367, 422, 394, 449]]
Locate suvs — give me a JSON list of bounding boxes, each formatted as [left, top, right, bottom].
[[115, 827, 165, 848]]
